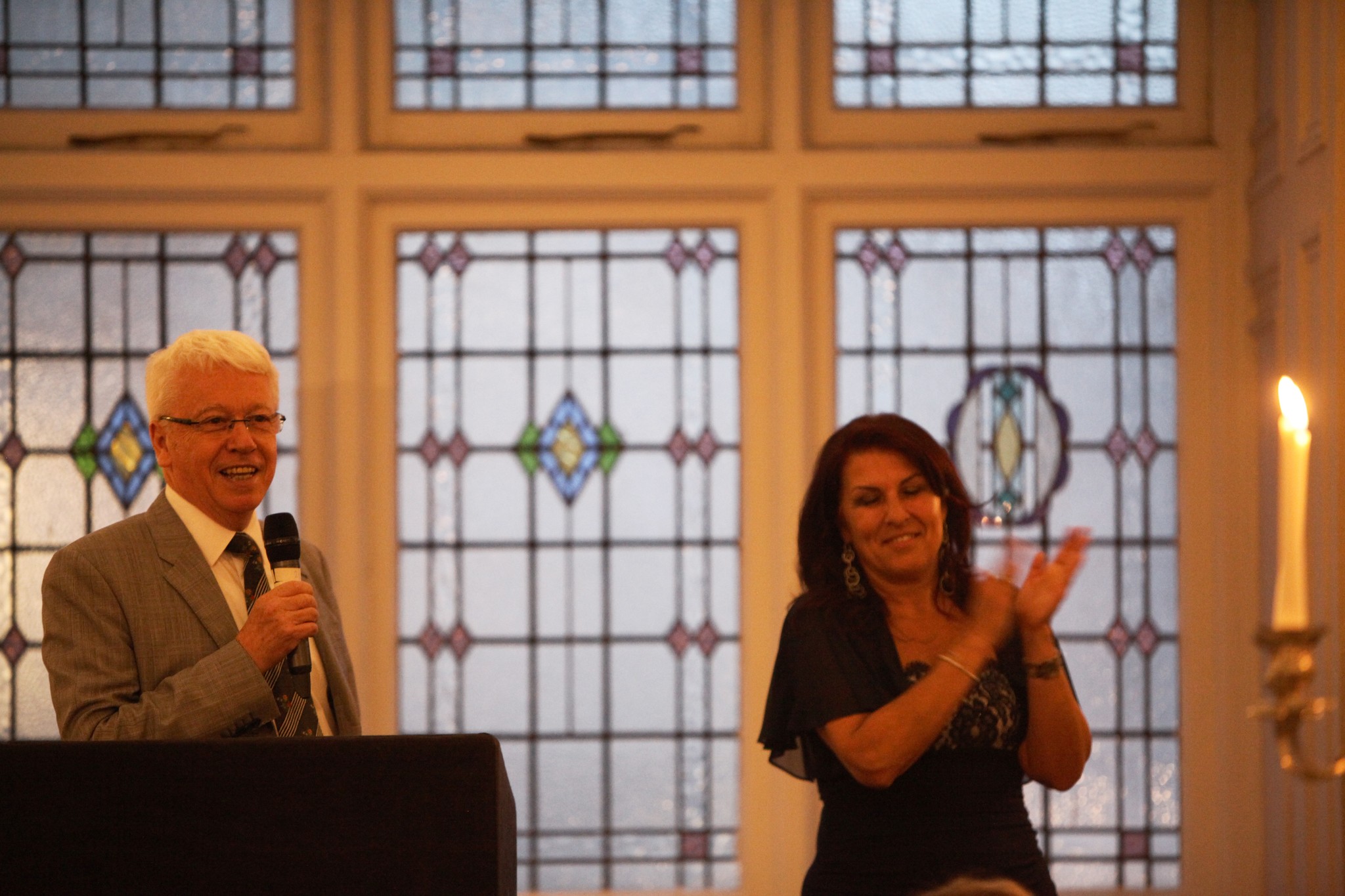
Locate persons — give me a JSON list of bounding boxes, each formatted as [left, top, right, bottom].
[[753, 412, 1092, 896], [40, 328, 362, 742]]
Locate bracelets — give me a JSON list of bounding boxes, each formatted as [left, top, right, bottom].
[[936, 653, 982, 684]]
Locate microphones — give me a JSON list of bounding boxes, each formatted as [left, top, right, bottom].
[[263, 511, 314, 700]]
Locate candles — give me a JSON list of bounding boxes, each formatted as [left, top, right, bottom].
[[1262, 372, 1315, 634]]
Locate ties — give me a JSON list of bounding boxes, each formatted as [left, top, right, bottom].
[[224, 531, 323, 737]]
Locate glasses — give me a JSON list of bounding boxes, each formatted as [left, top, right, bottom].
[[156, 412, 286, 434]]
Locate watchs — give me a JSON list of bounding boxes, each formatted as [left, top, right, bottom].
[[1022, 647, 1063, 679]]
